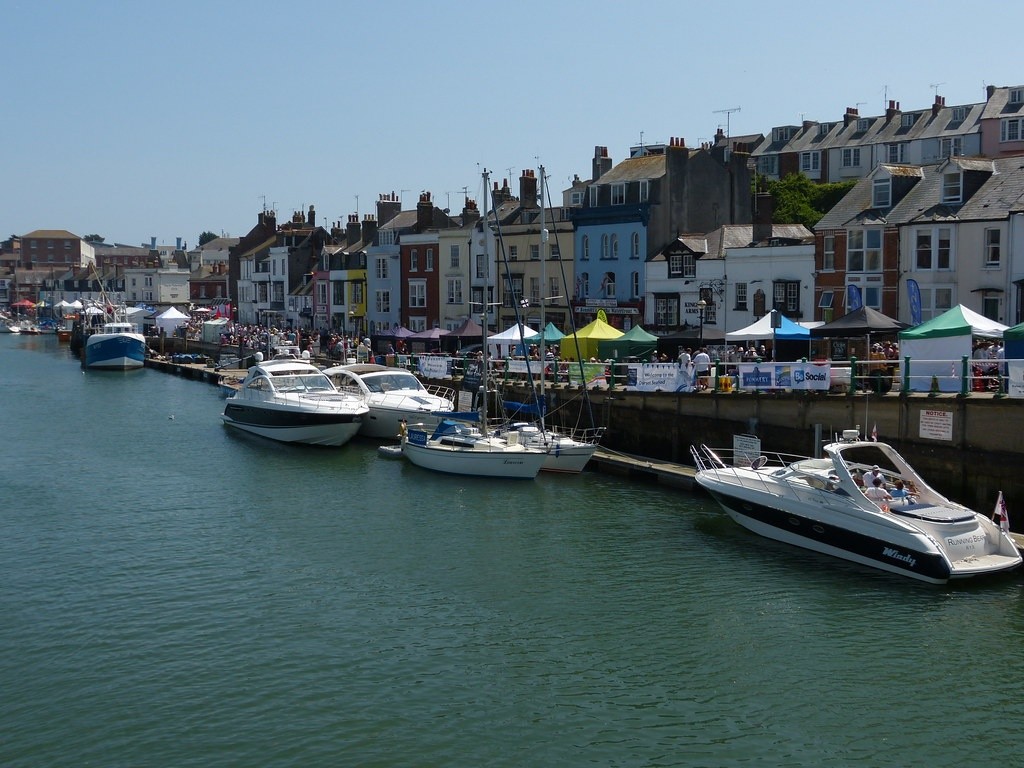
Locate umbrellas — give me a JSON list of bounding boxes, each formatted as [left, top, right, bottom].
[[193, 307, 210, 312]]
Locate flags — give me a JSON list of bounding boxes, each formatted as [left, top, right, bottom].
[[996, 493, 1015, 544]]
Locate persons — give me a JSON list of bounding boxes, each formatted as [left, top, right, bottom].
[[187, 312, 407, 364], [432, 344, 1004, 501]]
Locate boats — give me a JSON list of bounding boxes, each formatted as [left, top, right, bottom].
[[0, 318, 42, 336], [322, 363, 457, 441], [84, 322, 146, 372], [687, 423, 1023, 586], [220, 349, 371, 448]]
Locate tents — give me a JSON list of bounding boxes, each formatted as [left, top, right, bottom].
[[11, 299, 231, 344], [370, 305, 1024, 392]]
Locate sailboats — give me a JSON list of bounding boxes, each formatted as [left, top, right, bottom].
[[394, 163, 603, 481]]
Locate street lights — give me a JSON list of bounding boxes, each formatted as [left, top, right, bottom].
[[479, 312, 484, 354], [348, 310, 355, 348], [696, 296, 707, 346]]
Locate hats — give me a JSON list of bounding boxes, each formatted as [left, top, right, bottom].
[[893, 480, 903, 485], [738, 346, 744, 352], [749, 347, 756, 351], [872, 340, 898, 352], [760, 345, 765, 350], [873, 465, 880, 471]]
